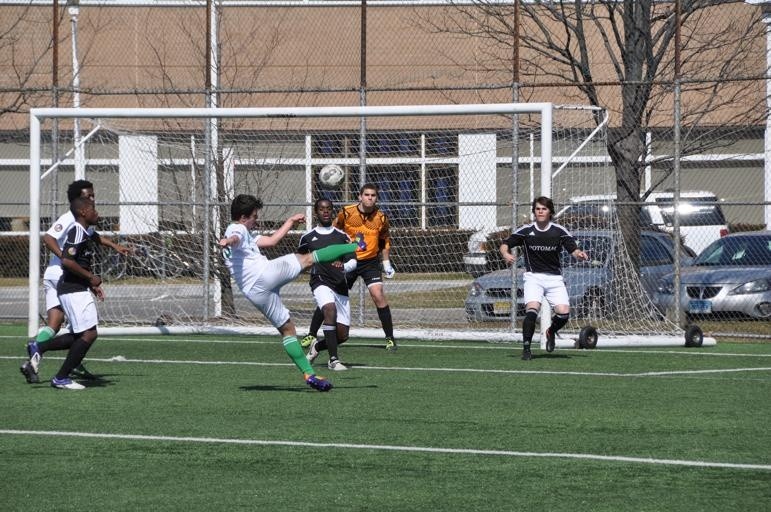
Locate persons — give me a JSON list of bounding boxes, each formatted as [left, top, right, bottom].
[[20, 179, 134, 384], [217, 194, 367, 391], [500, 197, 588, 360], [300, 183, 398, 351], [296, 197, 358, 371], [26, 196, 105, 390]]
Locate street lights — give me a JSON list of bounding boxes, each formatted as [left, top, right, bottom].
[[67, 1, 88, 181]]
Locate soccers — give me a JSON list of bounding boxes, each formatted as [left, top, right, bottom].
[[319, 165, 345, 189]]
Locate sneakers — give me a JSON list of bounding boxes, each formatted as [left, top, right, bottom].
[[386, 336, 396, 350], [301, 334, 348, 392], [522, 341, 531, 360], [52, 364, 94, 389], [354, 232, 366, 252], [21, 340, 41, 383], [546, 328, 555, 352]]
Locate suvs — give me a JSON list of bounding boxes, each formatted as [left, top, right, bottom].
[[465, 188, 730, 282]]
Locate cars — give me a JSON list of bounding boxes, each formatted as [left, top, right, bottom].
[[656, 229, 770, 326], [463, 229, 692, 322]]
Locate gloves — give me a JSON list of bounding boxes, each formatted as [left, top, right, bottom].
[[383, 260, 395, 279]]
